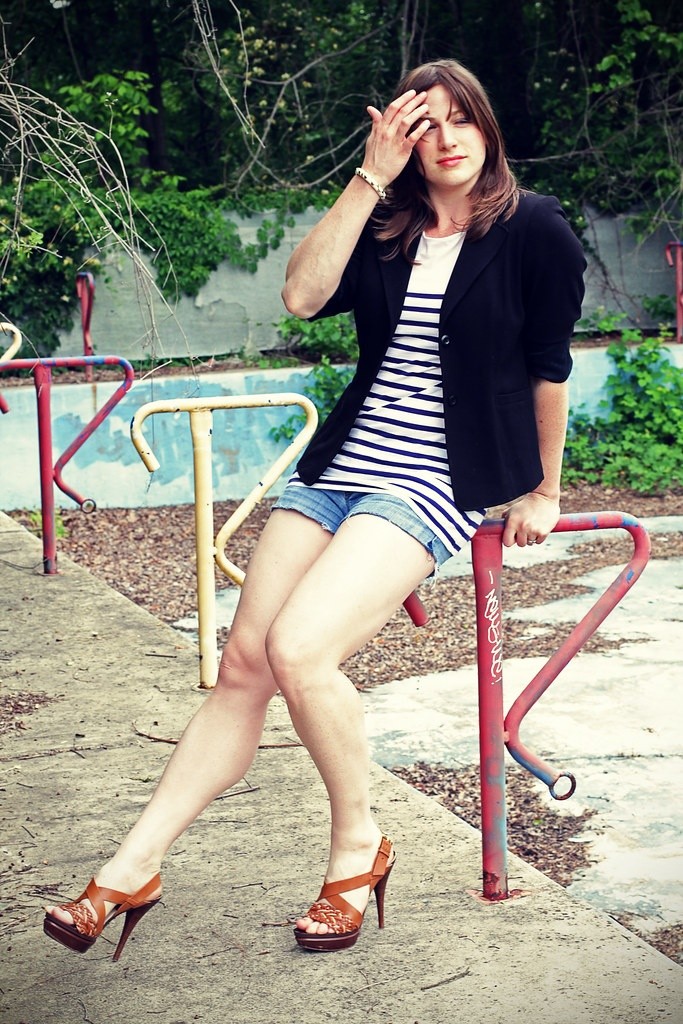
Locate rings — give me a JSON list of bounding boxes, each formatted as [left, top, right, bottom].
[[528, 540, 536, 543]]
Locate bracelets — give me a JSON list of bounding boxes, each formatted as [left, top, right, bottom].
[[355, 168, 386, 200]]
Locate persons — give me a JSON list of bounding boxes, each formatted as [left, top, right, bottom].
[[43, 60, 588, 963]]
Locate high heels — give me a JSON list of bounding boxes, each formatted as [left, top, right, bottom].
[[293, 833, 397, 951], [43, 871, 163, 961]]
